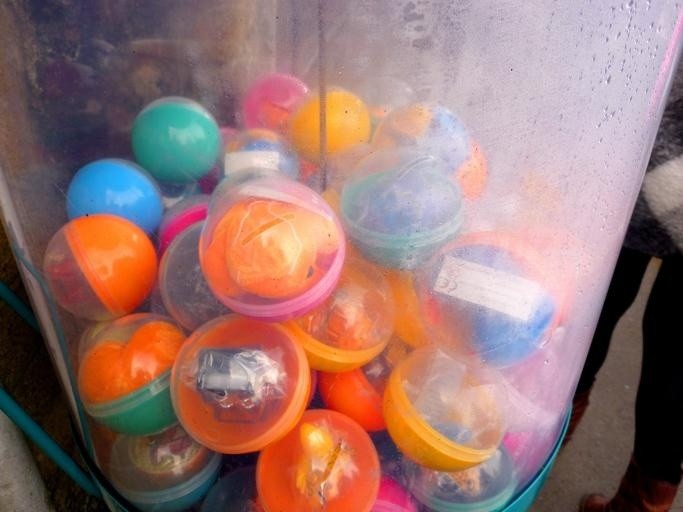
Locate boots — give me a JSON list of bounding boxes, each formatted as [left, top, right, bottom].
[[562, 401, 588, 444], [583, 455, 677, 512]]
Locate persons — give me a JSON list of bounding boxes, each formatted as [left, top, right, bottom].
[[560, 48, 683, 512]]
